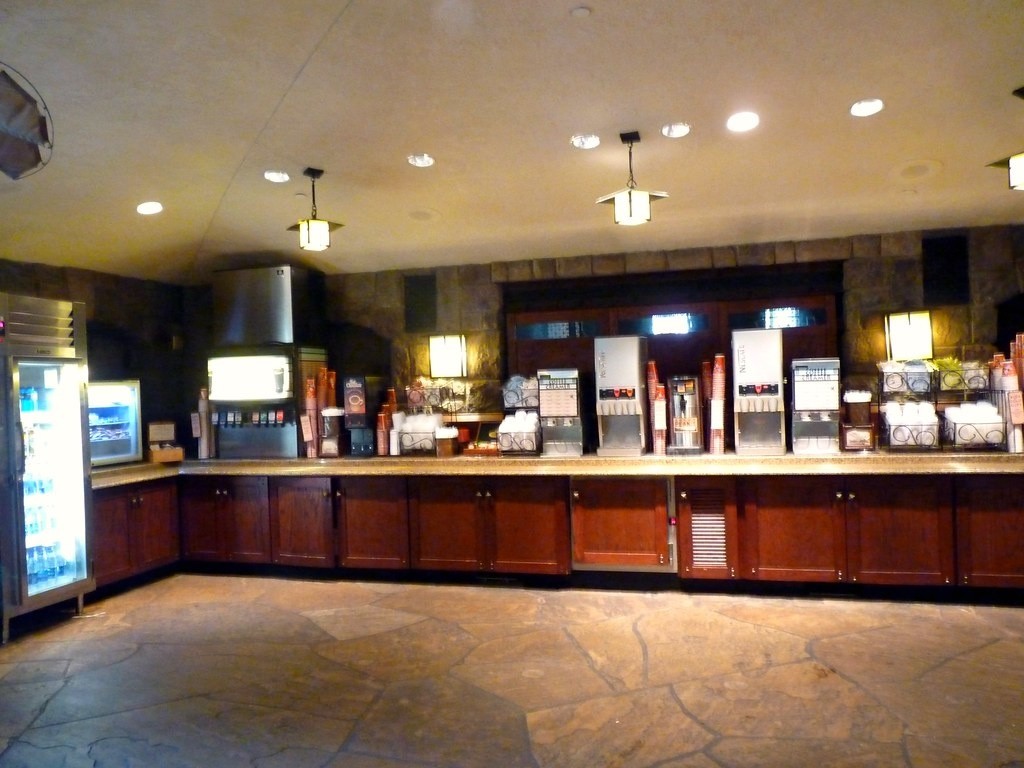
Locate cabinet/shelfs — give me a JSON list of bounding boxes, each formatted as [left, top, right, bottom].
[[93, 475, 1024, 590]]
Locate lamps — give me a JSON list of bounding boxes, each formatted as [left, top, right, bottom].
[[286, 169, 342, 250], [597, 130, 669, 225]]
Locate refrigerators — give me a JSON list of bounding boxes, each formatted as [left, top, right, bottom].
[[0, 354, 98, 645]]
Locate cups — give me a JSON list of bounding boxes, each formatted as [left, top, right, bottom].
[[701, 354, 727, 454], [305, 366, 337, 459], [647, 358, 666, 457], [197, 387, 215, 459], [987, 332, 1024, 453], [377, 386, 398, 456]]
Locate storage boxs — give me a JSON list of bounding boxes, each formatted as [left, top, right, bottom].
[[147, 443, 186, 464], [876, 359, 930, 393], [933, 358, 989, 389], [496, 413, 540, 453], [937, 409, 1007, 446], [148, 420, 174, 441], [840, 422, 876, 451], [500, 376, 540, 408], [881, 405, 939, 446]]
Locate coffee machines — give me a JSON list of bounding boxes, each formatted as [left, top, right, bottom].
[[343, 373, 391, 456]]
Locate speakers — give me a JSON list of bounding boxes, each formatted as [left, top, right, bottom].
[[922, 236, 969, 305], [403, 274, 437, 334]]
